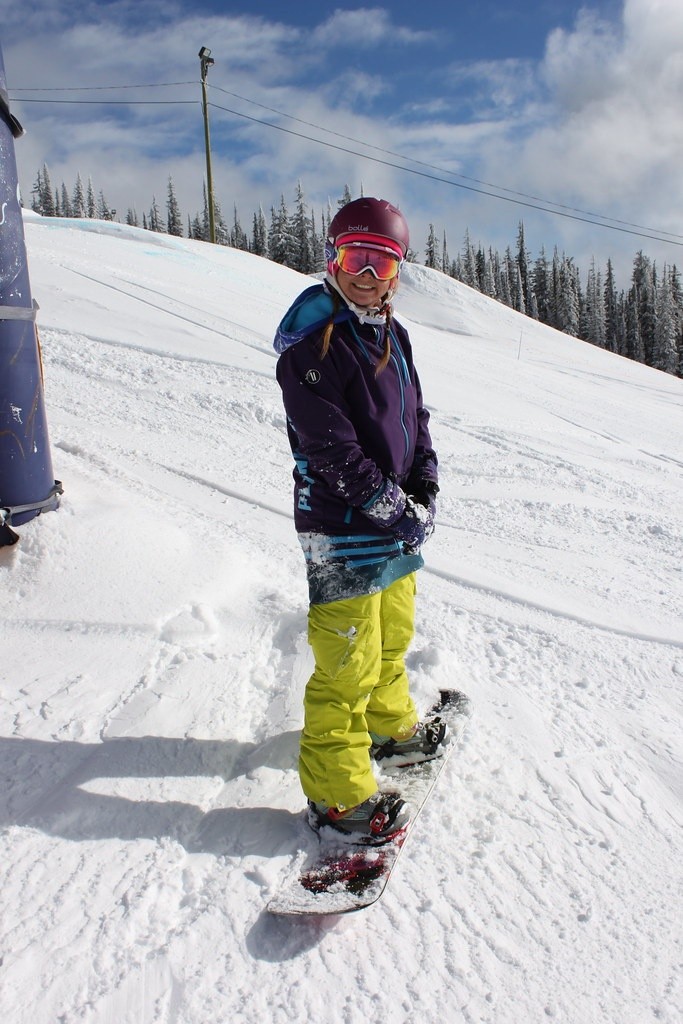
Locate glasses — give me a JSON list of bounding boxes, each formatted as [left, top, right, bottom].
[[337, 244, 402, 280]]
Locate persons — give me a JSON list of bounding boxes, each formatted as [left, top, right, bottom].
[[273, 197, 453, 848]]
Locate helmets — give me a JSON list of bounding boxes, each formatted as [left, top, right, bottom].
[[325, 197, 409, 263]]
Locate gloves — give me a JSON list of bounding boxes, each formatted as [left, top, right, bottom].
[[387, 497, 435, 557]]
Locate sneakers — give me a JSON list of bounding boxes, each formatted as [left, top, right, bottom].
[[369, 721, 450, 760], [307, 790, 410, 844]]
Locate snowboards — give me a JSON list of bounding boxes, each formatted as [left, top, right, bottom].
[[262, 678, 470, 925]]
